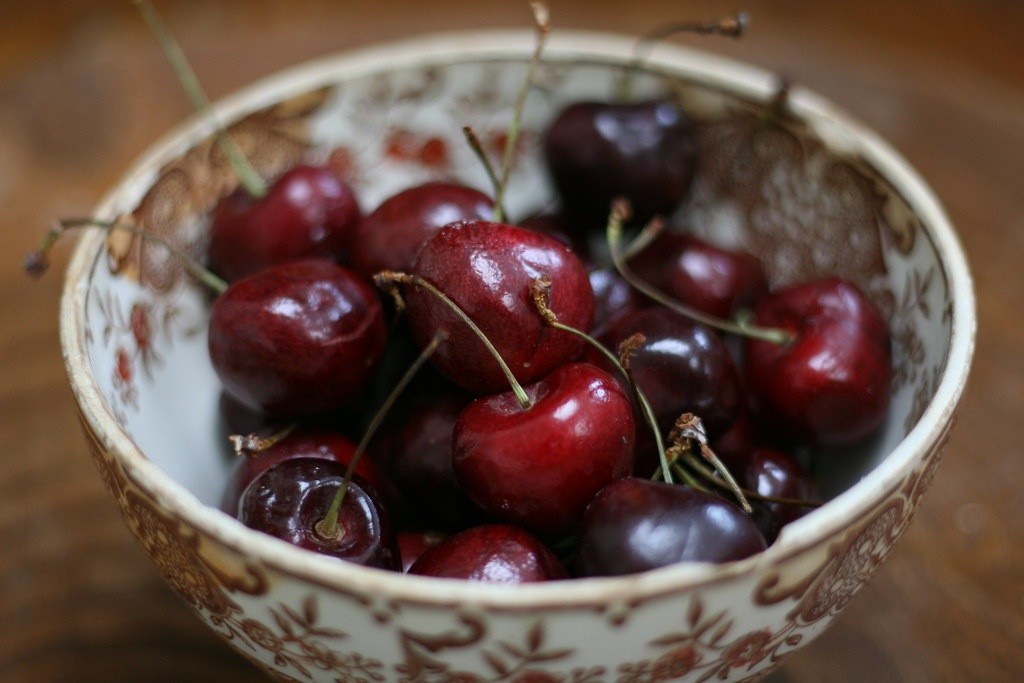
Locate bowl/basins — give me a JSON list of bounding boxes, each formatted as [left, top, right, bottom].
[[59, 28, 976, 683]]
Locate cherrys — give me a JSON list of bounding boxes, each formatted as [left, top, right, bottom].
[[17, 0, 897, 585]]
